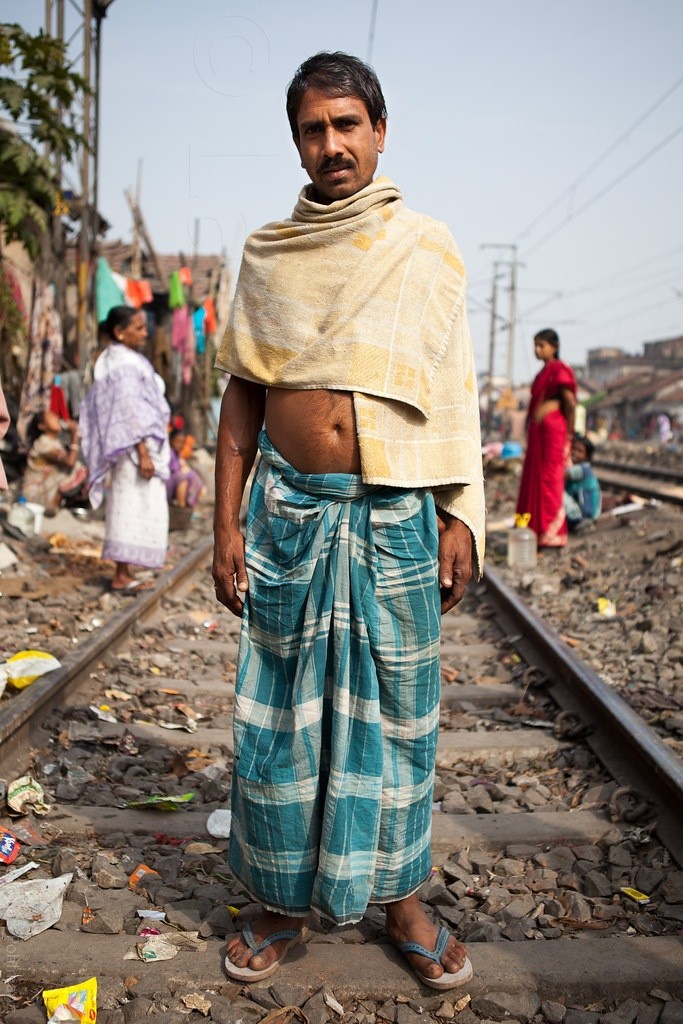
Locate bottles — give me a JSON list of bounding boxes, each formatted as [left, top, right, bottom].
[[507, 513, 538, 570]]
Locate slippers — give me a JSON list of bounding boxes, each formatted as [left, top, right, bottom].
[[386, 922, 473, 990], [224, 912, 310, 981], [111, 578, 154, 594]]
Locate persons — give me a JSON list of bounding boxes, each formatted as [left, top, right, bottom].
[[212, 52, 485, 990], [164, 428, 202, 507], [564, 438, 602, 525], [23, 411, 89, 511], [79, 304, 171, 592], [659, 412, 670, 444], [516, 329, 577, 547]]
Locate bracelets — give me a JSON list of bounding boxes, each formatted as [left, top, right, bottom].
[[69, 443, 79, 451]]
